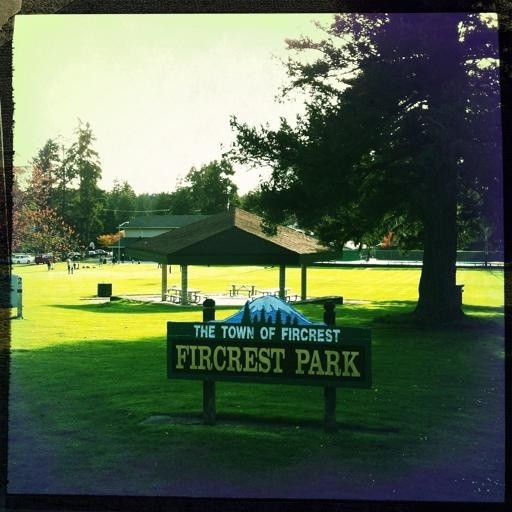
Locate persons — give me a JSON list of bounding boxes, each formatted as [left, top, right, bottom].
[[66, 256, 71, 274], [48, 259, 51, 270]]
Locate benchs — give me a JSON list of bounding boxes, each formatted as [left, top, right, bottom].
[[164, 284, 207, 306], [225, 283, 300, 304]]
